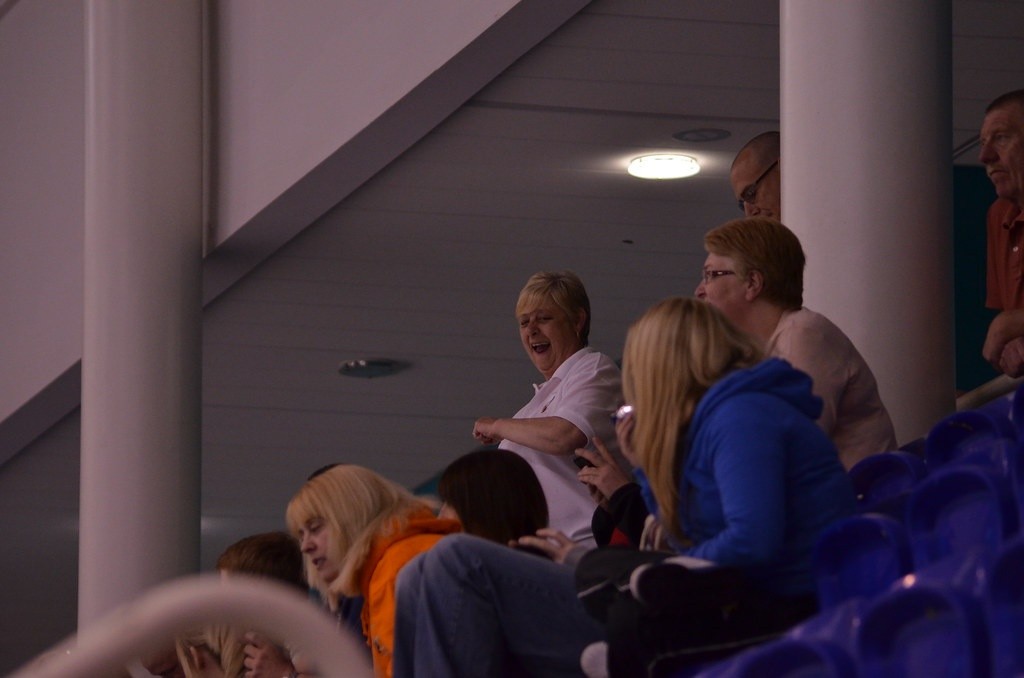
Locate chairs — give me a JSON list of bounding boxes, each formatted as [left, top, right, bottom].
[[631, 388, 1024, 678]]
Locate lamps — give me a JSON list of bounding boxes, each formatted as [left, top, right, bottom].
[[332, 351, 410, 378], [627, 153, 701, 180]]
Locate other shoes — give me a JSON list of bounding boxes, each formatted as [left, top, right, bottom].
[[629, 556, 736, 614], [580, 639, 612, 678]]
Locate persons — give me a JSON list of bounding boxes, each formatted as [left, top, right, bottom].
[[730, 130, 780, 223], [437, 450, 548, 558], [393, 297, 848, 678], [472, 273, 630, 550], [176, 461, 462, 678], [979, 90, 1024, 378], [695, 217, 898, 473]]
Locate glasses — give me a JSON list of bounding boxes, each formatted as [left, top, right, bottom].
[[738, 161, 778, 211], [702, 270, 736, 283]]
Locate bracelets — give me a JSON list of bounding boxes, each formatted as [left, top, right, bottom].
[[283, 672, 297, 678]]
